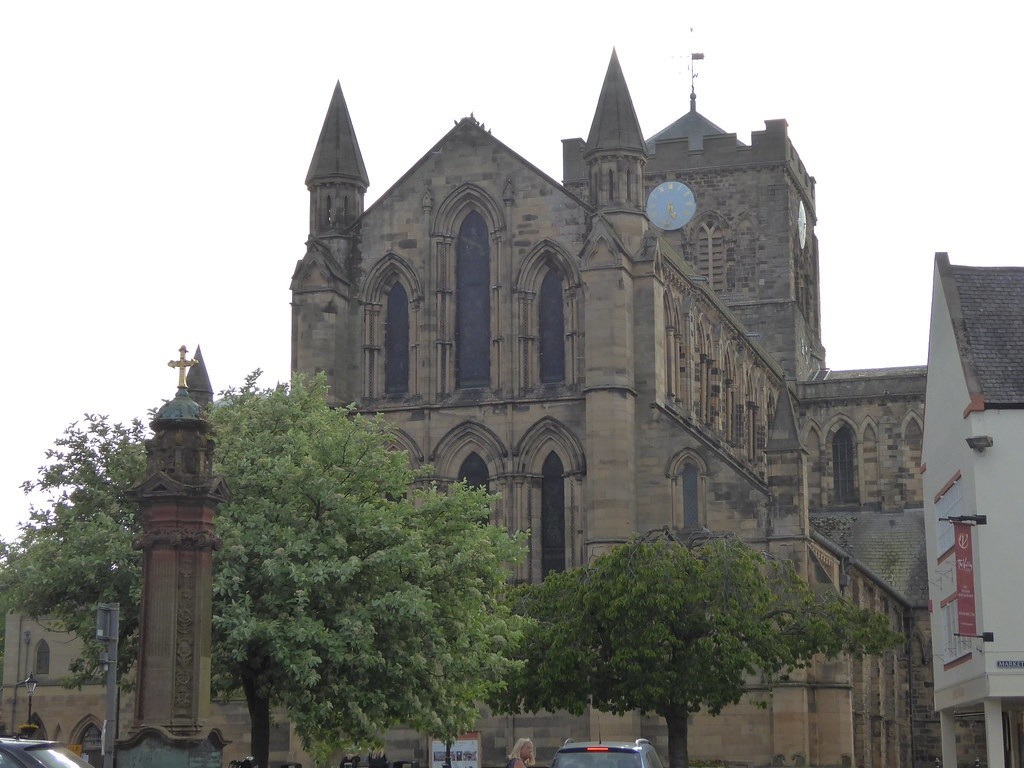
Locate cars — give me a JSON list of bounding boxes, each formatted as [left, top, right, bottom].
[[551, 736, 664, 768], [0, 736, 96, 768]]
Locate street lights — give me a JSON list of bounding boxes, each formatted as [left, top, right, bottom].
[[24, 673, 38, 738]]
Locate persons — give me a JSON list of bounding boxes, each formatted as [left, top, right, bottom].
[[505, 736, 536, 768], [340, 747, 388, 768]]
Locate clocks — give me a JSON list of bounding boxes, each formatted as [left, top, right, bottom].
[[646, 182, 696, 230]]
